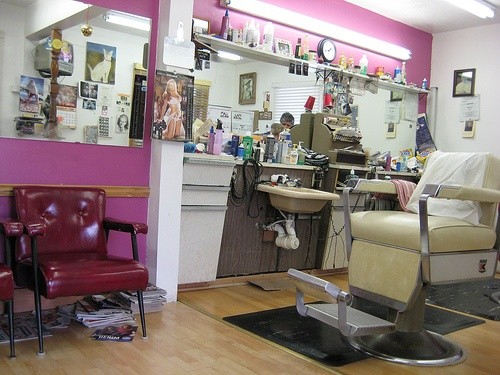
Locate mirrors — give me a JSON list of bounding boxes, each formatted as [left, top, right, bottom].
[[0, 0, 153, 148], [184, 39, 419, 171]]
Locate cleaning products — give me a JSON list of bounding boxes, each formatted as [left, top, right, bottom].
[[213, 119, 223, 155]]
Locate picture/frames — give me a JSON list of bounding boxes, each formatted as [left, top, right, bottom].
[[238, 72, 256, 104], [389, 91, 402, 101], [452, 69, 476, 97]]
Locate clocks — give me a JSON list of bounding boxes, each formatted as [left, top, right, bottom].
[[317, 39, 336, 63]]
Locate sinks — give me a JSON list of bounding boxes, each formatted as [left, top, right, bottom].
[[256, 184, 340, 214]]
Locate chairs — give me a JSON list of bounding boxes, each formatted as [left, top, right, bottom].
[[288, 151, 500, 369], [0, 185, 149, 359]]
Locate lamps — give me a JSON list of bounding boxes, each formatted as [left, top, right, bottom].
[[447, 0, 497, 18], [104, 8, 152, 32], [220, 0, 412, 62]]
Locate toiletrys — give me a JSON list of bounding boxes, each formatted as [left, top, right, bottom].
[[295, 37, 309, 61], [253, 128, 305, 165], [243, 131, 253, 161], [422, 78, 428, 89], [207, 126, 214, 154], [233, 20, 276, 51], [226, 141, 232, 154], [231, 135, 239, 156], [219, 9, 230, 40], [238, 140, 244, 160], [339, 51, 407, 84]]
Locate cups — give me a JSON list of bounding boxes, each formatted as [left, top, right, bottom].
[[304, 96, 315, 110], [324, 93, 334, 109]]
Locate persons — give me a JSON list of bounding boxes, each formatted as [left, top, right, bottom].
[[155, 78, 183, 139], [24, 79, 39, 108], [266, 112, 297, 137]]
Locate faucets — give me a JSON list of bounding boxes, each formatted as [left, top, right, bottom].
[[277, 176, 292, 183]]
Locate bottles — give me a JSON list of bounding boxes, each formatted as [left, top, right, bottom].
[[422, 78, 427, 90], [339, 51, 402, 83], [231, 129, 293, 165], [386, 153, 407, 173], [295, 34, 310, 60], [220, 9, 274, 53]]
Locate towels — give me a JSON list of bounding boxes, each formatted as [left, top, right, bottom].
[[302, 146, 329, 166], [374, 179, 417, 211]]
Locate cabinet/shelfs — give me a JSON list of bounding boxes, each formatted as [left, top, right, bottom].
[[178, 162, 422, 290]]
